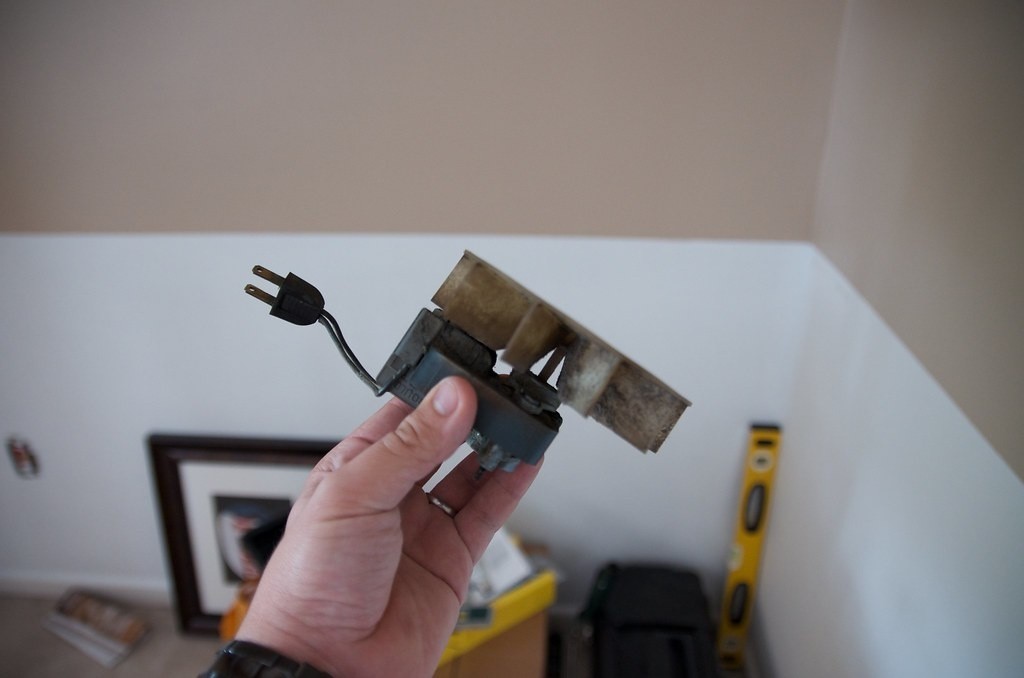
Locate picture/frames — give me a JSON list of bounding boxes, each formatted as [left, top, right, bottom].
[[143, 432, 341, 637]]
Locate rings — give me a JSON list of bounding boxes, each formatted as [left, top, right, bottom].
[[427, 491, 458, 519]]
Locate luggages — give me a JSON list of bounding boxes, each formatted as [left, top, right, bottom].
[[576, 562, 717, 676]]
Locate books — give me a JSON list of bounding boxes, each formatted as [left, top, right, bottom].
[[42, 583, 149, 673]]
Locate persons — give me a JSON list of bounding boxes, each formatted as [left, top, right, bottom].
[[192, 373, 547, 678]]
